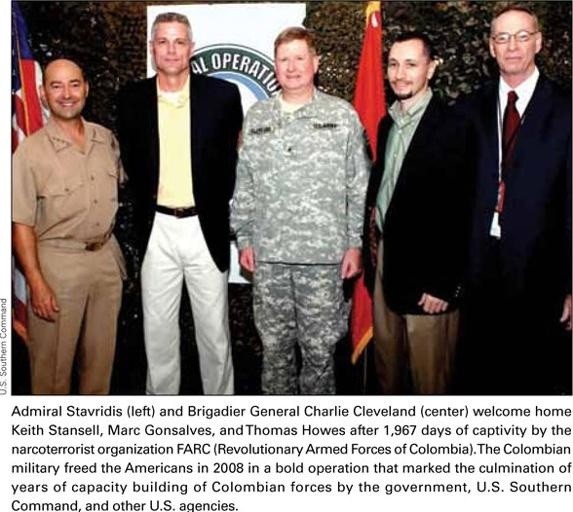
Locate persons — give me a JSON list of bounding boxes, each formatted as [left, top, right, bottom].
[[113, 12, 244, 395], [364, 30, 480, 393], [225, 25, 374, 394], [12, 58, 130, 395], [453, 7, 570, 396]]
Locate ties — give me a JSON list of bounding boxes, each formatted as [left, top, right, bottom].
[[494, 90, 520, 215]]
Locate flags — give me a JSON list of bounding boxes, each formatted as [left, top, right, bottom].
[[11, 5, 53, 351], [347, 1, 392, 368]]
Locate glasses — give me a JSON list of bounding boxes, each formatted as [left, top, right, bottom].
[[491, 29, 536, 44]]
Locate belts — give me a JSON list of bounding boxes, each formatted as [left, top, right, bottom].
[[155, 205, 196, 217], [50, 233, 111, 251]]
